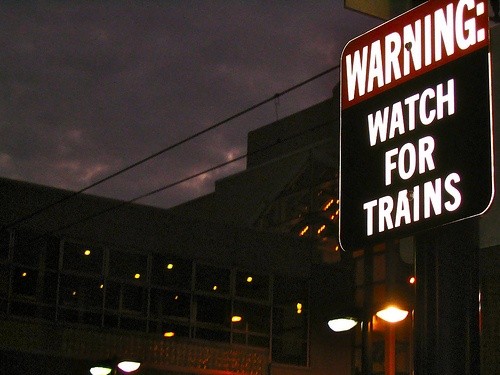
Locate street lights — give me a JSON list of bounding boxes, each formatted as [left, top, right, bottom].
[[375, 296, 412, 375], [87, 352, 142, 375]]
